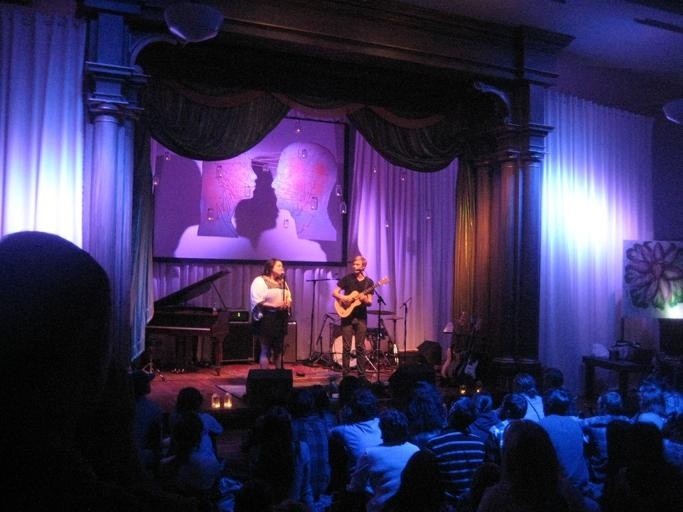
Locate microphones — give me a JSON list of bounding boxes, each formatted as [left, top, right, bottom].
[[325, 312, 336, 323], [399, 297, 412, 309], [356, 269, 364, 272]]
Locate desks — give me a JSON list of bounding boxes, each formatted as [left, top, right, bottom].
[[583, 355, 642, 407]]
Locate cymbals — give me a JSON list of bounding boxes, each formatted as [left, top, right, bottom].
[[367, 310, 397, 315], [387, 316, 402, 320]]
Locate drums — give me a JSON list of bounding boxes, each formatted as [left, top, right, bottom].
[[333, 336, 371, 368]]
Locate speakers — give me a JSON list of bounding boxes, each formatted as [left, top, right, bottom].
[[245, 369, 294, 405]]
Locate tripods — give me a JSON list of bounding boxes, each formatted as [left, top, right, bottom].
[[367, 334, 391, 370], [311, 336, 332, 368]]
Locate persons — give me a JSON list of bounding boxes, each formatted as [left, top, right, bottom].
[[130, 369, 683, 511], [0, 232, 200, 512], [250, 259, 292, 370], [332, 256, 374, 378]]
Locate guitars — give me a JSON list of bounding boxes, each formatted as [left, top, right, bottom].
[[334, 276, 390, 318]]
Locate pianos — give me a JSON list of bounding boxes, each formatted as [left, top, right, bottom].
[[144, 271, 230, 375]]
